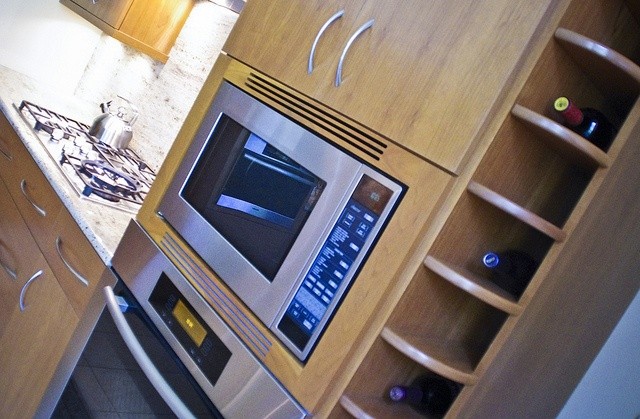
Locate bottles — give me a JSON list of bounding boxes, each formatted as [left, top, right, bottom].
[[387, 370, 465, 418], [482, 242, 545, 301], [553, 95, 623, 154]]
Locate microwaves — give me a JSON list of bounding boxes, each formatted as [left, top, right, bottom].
[[156, 76, 404, 369]]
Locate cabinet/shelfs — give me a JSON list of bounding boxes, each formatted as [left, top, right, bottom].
[[57, 0, 200, 64], [315, 0, 639, 418], [222, 0, 553, 172], [0, 108, 115, 418]]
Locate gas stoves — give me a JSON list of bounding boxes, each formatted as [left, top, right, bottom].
[[10, 99, 158, 215]]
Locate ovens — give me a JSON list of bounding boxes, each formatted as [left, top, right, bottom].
[[26, 217, 317, 419], [216, 131, 320, 230]]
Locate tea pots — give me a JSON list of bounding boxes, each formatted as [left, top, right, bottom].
[[86, 93, 142, 152]]
